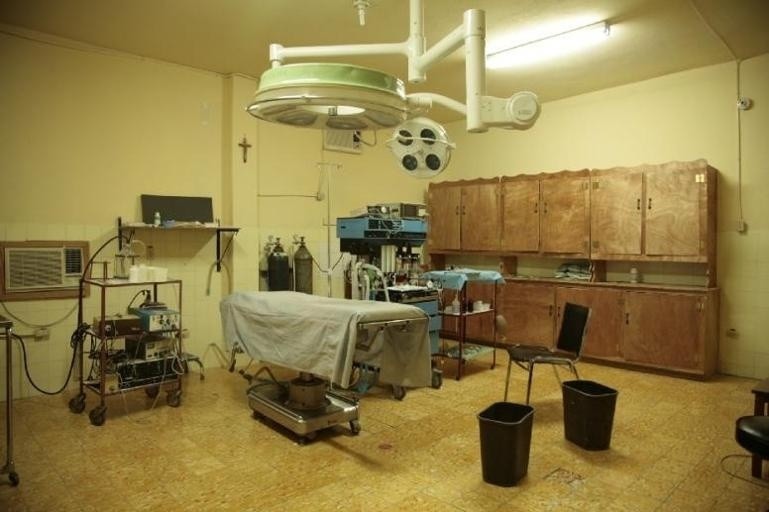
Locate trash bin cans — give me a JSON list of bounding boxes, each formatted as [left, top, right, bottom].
[[477, 401, 535, 487], [561, 379, 618, 450]]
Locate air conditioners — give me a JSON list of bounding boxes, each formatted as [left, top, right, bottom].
[[1, 239, 93, 300]]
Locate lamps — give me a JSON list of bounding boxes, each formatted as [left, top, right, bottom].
[[242, 2, 542, 180]]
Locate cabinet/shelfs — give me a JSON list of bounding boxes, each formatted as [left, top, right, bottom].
[[589, 157, 718, 263], [425, 177, 500, 256], [501, 168, 589, 259], [442, 279, 496, 341], [495, 278, 620, 362], [413, 267, 505, 381], [622, 284, 720, 380]]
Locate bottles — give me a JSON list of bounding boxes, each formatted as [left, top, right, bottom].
[[154, 212, 161, 226], [629, 266, 640, 283]]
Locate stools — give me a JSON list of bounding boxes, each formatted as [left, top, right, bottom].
[[730, 381, 768, 480]]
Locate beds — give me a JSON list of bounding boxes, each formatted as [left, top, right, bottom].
[[217, 287, 435, 448]]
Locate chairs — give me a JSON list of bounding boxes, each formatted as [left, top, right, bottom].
[[502, 300, 593, 407]]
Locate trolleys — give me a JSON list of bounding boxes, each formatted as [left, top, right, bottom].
[[423, 267, 499, 382]]
